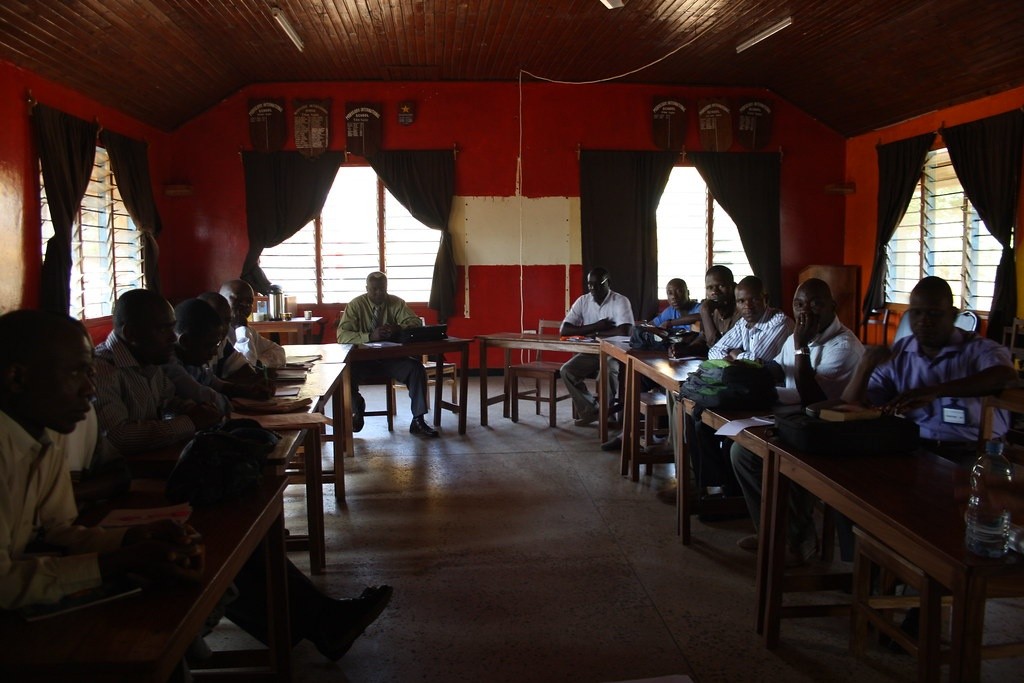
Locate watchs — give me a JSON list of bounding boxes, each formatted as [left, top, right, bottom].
[[666, 319, 672, 328], [795, 346, 810, 356], [595, 330, 599, 336]]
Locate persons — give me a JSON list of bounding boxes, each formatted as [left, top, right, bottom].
[[336, 272, 440, 436], [0, 280, 392, 663], [560, 265, 1024, 652]]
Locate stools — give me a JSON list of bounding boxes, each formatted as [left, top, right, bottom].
[[850, 527, 985, 683], [637, 392, 669, 476]]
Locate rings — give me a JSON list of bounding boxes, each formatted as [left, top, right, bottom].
[[799, 321, 805, 325]]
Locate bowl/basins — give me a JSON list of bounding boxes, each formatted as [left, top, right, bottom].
[[252, 313, 265, 322]]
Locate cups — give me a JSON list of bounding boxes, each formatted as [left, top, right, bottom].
[[303, 310, 312, 320], [282, 313, 292, 321]]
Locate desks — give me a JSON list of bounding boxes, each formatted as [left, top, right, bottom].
[[0, 317, 1024, 683]]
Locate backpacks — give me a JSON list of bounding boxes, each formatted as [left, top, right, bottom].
[[630, 325, 688, 351], [676, 359, 778, 421], [388, 324, 449, 343]]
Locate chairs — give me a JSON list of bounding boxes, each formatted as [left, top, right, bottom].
[[1008, 317, 1024, 371], [953, 311, 979, 333], [861, 310, 889, 351], [335, 311, 397, 432], [417, 316, 457, 414], [509, 319, 574, 426]]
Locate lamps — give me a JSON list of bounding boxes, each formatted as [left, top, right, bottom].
[[272, 7, 304, 52], [735, 16, 793, 53]]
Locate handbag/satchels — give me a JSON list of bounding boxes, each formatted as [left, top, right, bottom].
[[390, 324, 449, 344], [773, 411, 920, 455], [173, 419, 278, 510]]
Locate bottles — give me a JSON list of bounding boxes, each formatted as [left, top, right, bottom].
[[963, 438, 1013, 558]]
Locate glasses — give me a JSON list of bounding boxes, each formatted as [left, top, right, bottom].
[[185, 336, 224, 351], [587, 278, 608, 286]]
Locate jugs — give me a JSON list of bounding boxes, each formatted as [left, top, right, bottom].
[[266, 285, 285, 321]]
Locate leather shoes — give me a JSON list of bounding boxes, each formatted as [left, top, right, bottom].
[[352, 403, 365, 432], [410, 418, 438, 437]]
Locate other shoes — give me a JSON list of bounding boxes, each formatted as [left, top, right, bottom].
[[790, 542, 817, 565], [701, 505, 738, 520], [601, 438, 621, 449], [738, 534, 757, 553], [319, 585, 392, 661], [575, 415, 598, 426], [650, 447, 674, 462]]
